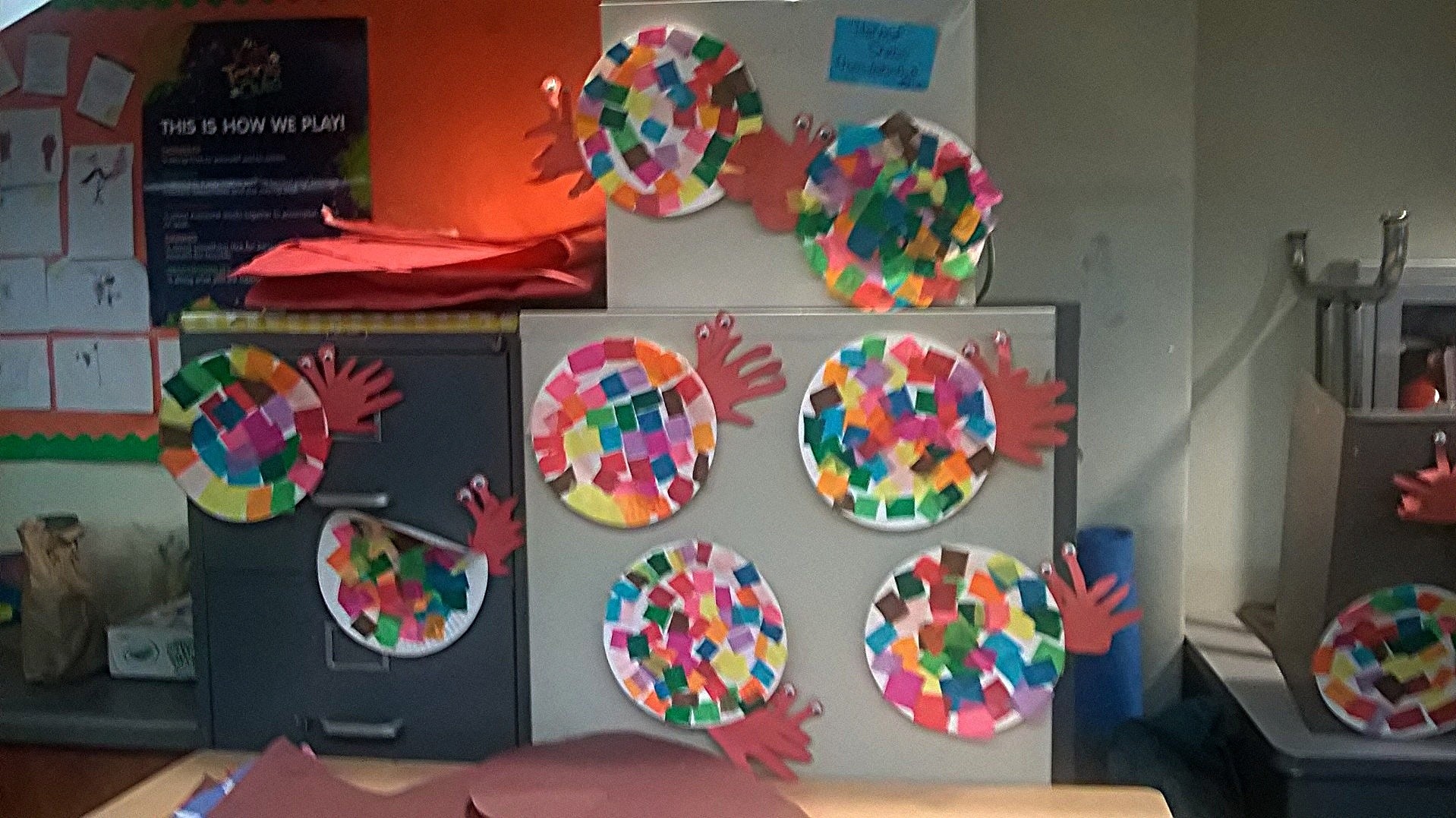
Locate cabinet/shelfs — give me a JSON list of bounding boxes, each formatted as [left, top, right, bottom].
[[182, 313, 517, 764]]
